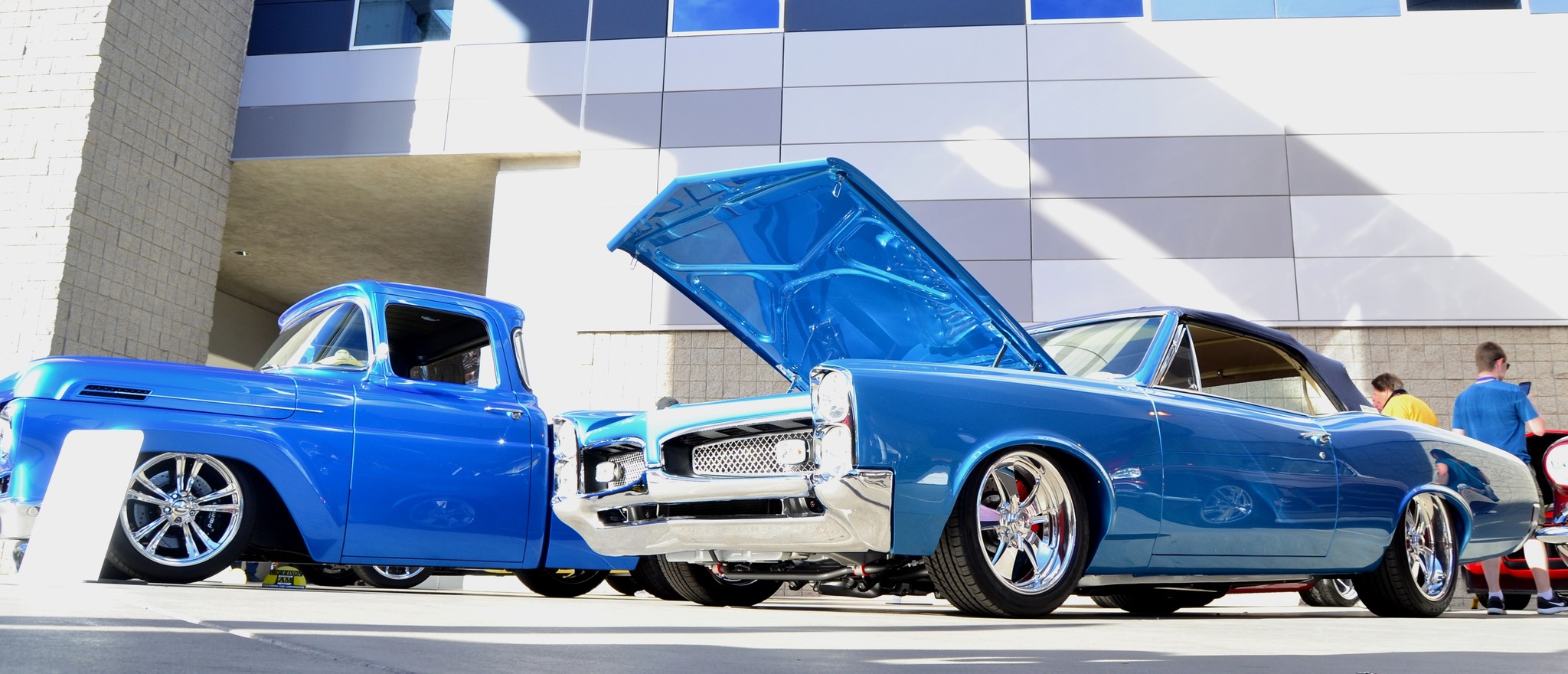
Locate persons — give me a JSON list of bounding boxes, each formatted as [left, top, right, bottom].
[[1370, 372, 1439, 428], [655, 396, 679, 410], [1371, 389, 1384, 413], [1430, 448, 1500, 514], [1449, 341, 1568, 614], [230, 561, 263, 582]]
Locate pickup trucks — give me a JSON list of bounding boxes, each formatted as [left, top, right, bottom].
[[3, 276, 791, 611]]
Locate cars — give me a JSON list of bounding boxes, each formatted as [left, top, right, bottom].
[[553, 156, 1546, 633]]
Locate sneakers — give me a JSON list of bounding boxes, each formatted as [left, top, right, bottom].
[[1486, 595, 1507, 615], [1536, 590, 1567, 614]]
